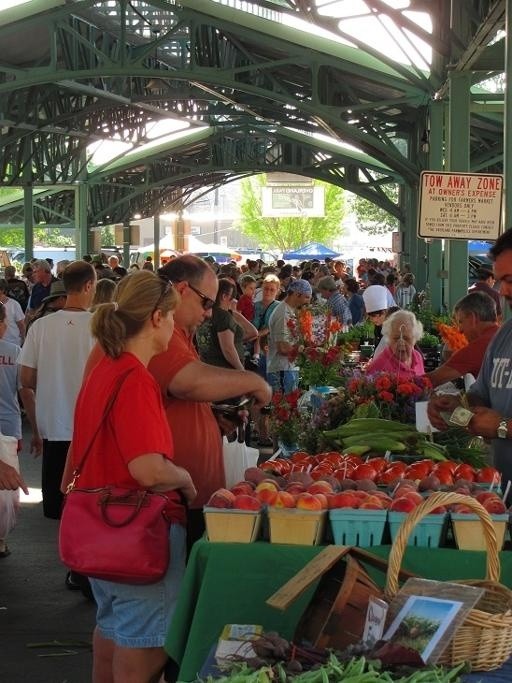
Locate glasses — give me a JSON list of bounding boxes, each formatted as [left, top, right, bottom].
[[177, 278, 217, 311], [151, 273, 173, 321]]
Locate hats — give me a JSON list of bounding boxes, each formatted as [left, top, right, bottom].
[[41, 280, 68, 302], [362, 283, 398, 314]]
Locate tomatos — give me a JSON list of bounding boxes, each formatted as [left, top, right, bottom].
[[259, 451, 501, 485]]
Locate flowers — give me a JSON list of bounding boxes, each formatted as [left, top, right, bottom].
[[346, 373, 432, 417], [286, 304, 351, 385], [265, 388, 312, 450]]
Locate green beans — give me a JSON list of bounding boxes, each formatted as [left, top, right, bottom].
[[196, 652, 466, 683]]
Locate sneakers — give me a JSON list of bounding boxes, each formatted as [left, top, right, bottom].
[[250, 354, 261, 367], [65, 570, 93, 601]]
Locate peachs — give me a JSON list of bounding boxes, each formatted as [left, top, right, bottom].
[[207, 467, 506, 515]]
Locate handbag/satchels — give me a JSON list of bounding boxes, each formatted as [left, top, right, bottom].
[[57, 484, 172, 586]]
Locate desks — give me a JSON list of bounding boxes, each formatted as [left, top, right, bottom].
[[192, 638, 512, 683], [165, 531, 512, 682]]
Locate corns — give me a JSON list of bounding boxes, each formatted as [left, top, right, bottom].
[[323, 400, 448, 461]]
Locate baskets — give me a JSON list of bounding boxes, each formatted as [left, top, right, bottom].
[[383, 490, 512, 672]]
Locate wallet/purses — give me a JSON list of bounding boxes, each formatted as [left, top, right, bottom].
[[210, 394, 256, 428]]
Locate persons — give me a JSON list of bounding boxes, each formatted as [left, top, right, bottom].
[[1, 227, 511, 683]]
